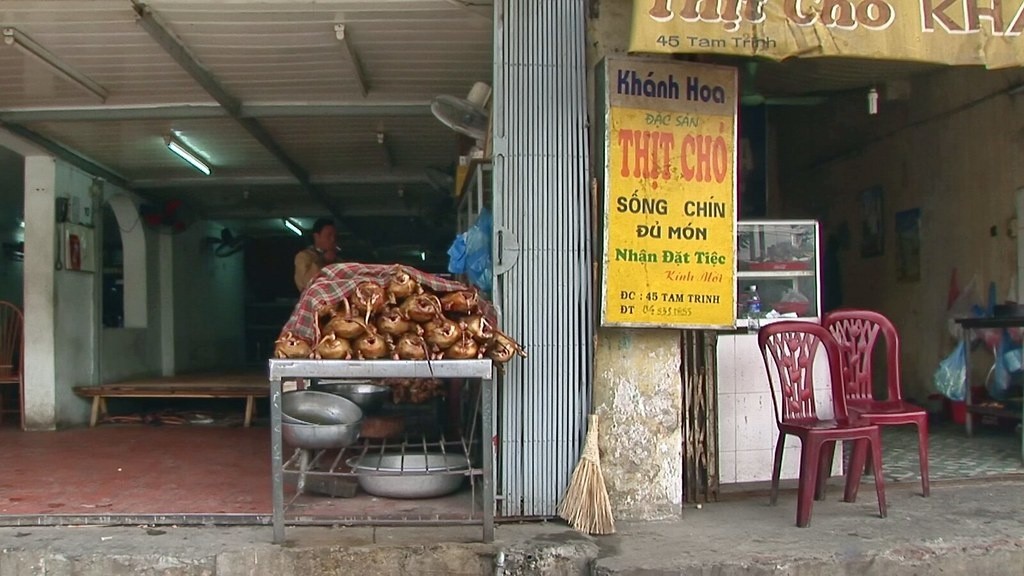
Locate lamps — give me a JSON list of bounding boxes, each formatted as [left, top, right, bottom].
[[161, 130, 212, 178], [335, 24, 369, 97], [377, 133, 393, 174], [2, 27, 109, 102]]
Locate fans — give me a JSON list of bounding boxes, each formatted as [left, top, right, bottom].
[[431, 81, 492, 158], [206, 228, 251, 258], [739, 61, 828, 107], [423, 166, 456, 196]]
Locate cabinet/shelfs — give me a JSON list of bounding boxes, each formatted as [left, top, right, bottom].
[[268, 357, 494, 543], [955, 318, 1024, 437], [456, 162, 493, 236], [736, 220, 822, 327]]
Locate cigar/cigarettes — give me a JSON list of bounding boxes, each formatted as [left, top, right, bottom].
[[335, 246, 341, 251]]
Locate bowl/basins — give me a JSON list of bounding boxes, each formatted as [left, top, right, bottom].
[[281, 383, 405, 450], [345, 450, 475, 499], [772, 301, 811, 316]]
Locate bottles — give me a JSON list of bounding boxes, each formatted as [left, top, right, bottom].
[[746, 285, 762, 333]]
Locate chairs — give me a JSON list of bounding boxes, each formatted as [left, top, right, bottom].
[[0, 301, 26, 429], [757, 321, 888, 527], [824, 308, 930, 503]]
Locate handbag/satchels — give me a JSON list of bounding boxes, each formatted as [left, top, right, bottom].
[[931, 338, 968, 401], [993, 328, 1024, 397], [448, 207, 494, 289]]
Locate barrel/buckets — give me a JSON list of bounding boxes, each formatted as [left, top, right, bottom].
[[949, 387, 985, 422]]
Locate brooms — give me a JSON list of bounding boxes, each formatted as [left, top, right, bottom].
[[558, 411, 623, 535]]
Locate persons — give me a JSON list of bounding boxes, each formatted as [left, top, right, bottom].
[[294, 218, 346, 293]]
